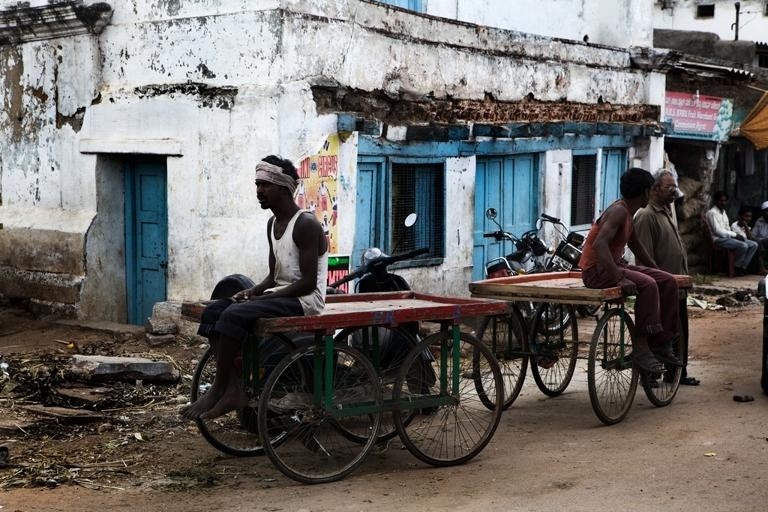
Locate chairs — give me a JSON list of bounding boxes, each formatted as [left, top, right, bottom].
[[695, 214, 734, 276]]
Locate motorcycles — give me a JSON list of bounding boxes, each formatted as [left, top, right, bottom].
[[260, 212, 436, 414], [483, 207, 601, 335]]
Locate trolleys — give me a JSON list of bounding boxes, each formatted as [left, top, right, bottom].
[[470, 269, 692, 424], [179, 291, 514, 483]]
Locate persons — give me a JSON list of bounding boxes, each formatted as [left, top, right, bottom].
[[633, 168, 700, 388], [179, 155, 328, 421], [578, 167, 684, 374], [705, 190, 759, 276], [730, 206, 768, 275], [752, 200, 768, 251]]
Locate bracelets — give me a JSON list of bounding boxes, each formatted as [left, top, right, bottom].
[[616, 276, 624, 283]]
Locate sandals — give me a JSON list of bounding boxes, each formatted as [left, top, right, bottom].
[[629, 349, 668, 374], [664, 374, 702, 385], [639, 378, 659, 389], [653, 346, 684, 368]]
